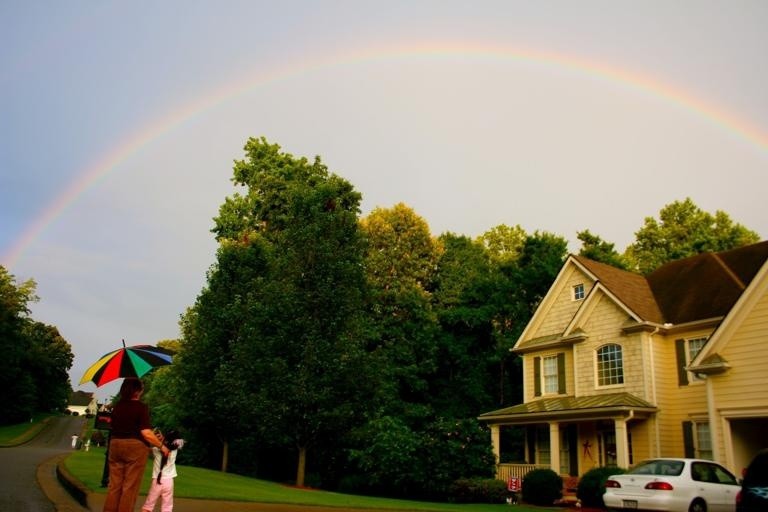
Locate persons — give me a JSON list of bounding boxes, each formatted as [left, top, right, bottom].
[[141, 427, 186, 511], [102, 377, 171, 511], [70, 433, 79, 448]]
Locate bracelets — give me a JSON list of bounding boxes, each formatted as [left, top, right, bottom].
[[157, 443, 164, 449]]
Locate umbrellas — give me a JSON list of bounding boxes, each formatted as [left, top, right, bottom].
[[77, 337, 177, 388]]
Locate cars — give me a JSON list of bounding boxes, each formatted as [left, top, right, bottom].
[[602, 457, 742, 512]]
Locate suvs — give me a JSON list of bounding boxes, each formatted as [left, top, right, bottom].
[[735, 449, 768, 511]]
